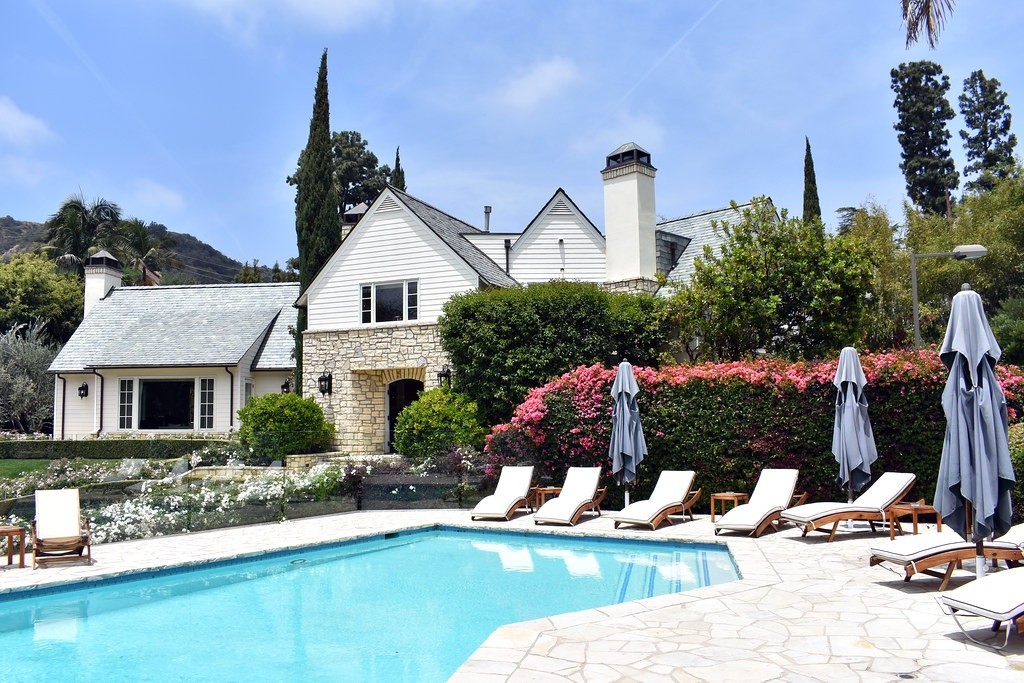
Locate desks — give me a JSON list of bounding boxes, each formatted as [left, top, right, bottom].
[[890, 505, 942, 540], [535, 488, 562, 511], [0, 525, 25, 568], [710, 493, 748, 522]]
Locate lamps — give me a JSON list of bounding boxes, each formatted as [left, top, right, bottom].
[[437, 365, 451, 390], [317, 372, 333, 398], [78, 382, 88, 399], [281, 379, 289, 394]]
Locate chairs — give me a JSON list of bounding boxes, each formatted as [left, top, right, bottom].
[[869, 522, 1024, 592], [533, 466, 609, 526], [614, 469, 702, 532], [941, 566, 1024, 650], [471, 465, 537, 522], [779, 472, 925, 543], [713, 468, 808, 539], [29, 488, 92, 570]]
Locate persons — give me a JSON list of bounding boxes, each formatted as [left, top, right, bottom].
[[144, 395, 164, 428]]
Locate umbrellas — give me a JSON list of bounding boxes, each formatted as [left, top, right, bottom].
[[829, 344, 879, 506], [608, 357, 649, 512], [931, 290, 1019, 582]]
